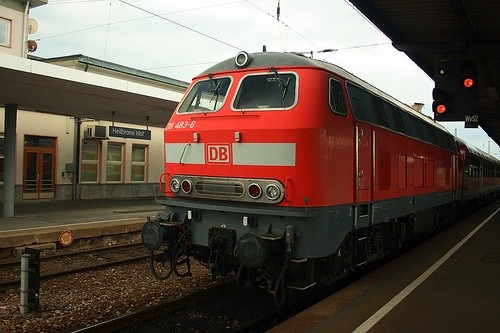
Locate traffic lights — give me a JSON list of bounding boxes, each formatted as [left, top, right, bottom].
[[432, 44, 480, 122]]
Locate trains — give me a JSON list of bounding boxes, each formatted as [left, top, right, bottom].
[[145, 51, 500, 299]]
